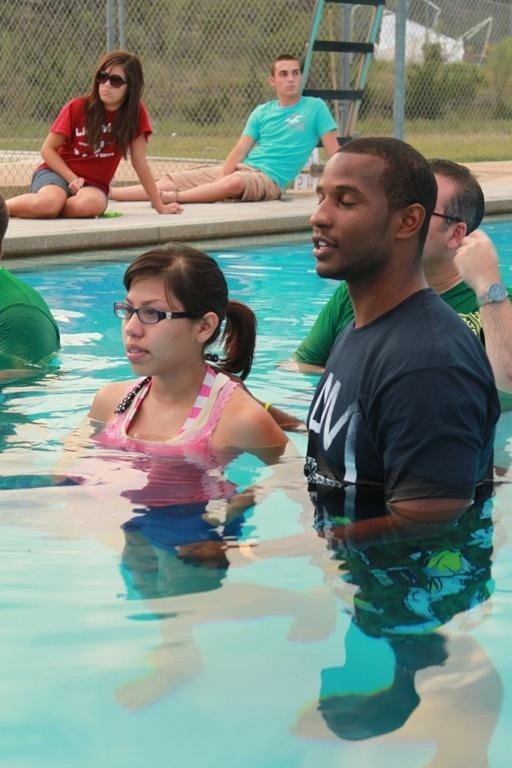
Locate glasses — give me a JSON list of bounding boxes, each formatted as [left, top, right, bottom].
[[111, 302, 205, 325], [96, 70, 130, 88]]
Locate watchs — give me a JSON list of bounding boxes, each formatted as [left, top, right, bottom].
[[475, 280, 509, 308]]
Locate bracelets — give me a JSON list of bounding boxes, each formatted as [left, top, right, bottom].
[[264, 401, 272, 412], [241, 540, 261, 562]]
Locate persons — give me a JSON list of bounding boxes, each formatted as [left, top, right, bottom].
[[0, 192, 64, 393], [51, 236, 339, 715], [108, 54, 345, 205], [170, 135, 502, 768], [274, 157, 511, 416], [4, 49, 186, 219]]
